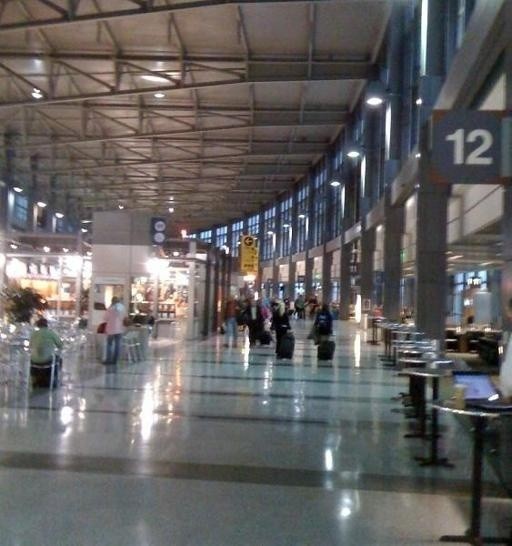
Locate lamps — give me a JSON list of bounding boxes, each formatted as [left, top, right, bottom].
[[284, 80, 406, 227]]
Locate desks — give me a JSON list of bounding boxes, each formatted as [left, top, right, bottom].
[[366, 314, 512, 546]]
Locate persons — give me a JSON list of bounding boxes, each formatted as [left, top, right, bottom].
[[221, 289, 339, 354], [497, 288, 512, 496], [102, 296, 126, 364], [27, 318, 65, 385]]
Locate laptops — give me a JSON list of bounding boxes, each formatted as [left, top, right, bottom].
[[452, 370, 512, 411]]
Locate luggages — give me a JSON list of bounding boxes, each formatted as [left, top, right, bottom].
[[260, 330, 271, 346], [317, 339, 336, 361], [274, 332, 296, 360]]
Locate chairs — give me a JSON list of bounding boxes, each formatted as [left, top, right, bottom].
[[0, 314, 150, 411]]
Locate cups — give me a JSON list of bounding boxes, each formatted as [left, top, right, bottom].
[[426, 353, 436, 369], [453, 383, 468, 410]]
